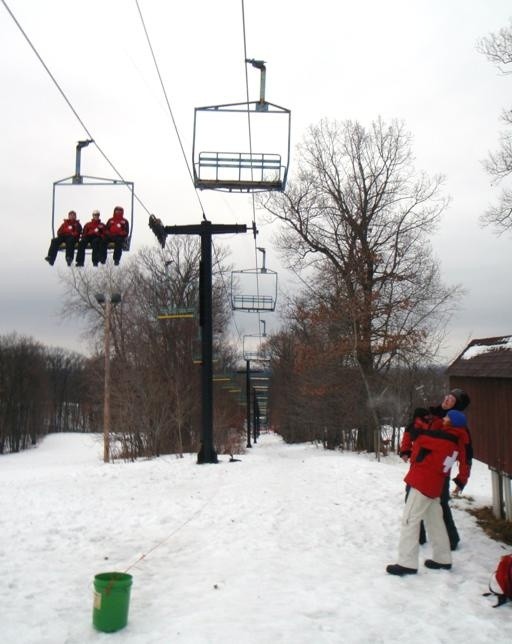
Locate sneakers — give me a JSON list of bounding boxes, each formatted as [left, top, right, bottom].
[[424, 560, 452, 571], [387, 564, 418, 575], [44, 257, 119, 266]]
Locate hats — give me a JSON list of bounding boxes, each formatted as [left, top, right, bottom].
[[443, 388, 470, 410], [447, 410, 467, 427]]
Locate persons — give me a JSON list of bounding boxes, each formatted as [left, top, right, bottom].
[[45, 206, 129, 266], [387, 389, 473, 575]]
